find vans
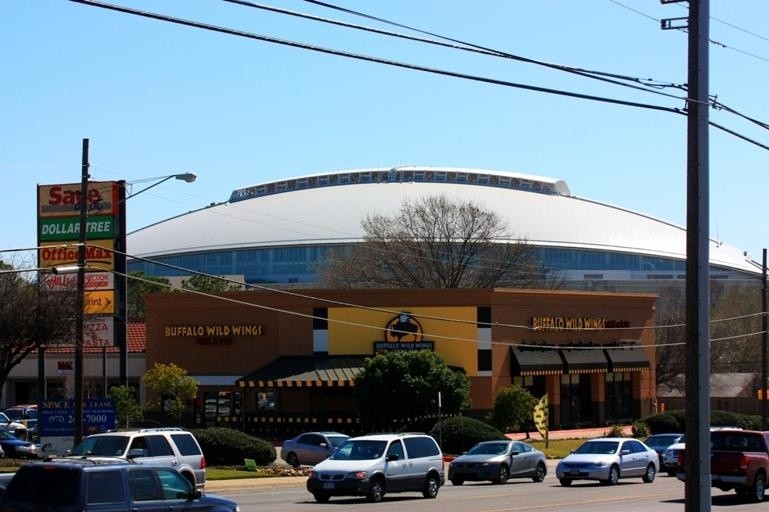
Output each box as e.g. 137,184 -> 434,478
49,426 -> 206,498
662,426 -> 744,477
0,462 -> 240,512
305,432 -> 445,503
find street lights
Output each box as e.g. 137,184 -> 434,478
73,137 -> 197,451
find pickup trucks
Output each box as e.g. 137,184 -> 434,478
675,428 -> 769,502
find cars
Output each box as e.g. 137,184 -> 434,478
0,405 -> 38,459
280,431 -> 352,467
556,437 -> 659,487
643,433 -> 684,467
448,440 -> 549,485
